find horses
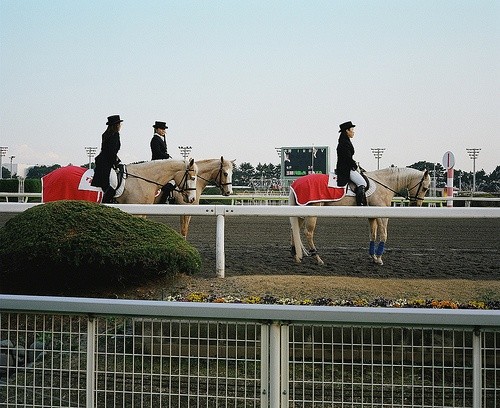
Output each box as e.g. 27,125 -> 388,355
288,167 -> 431,265
41,158 -> 198,218
152,156 -> 236,241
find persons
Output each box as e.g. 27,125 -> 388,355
91,115 -> 123,204
150,121 -> 173,204
336,121 -> 368,206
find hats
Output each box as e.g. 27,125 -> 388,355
338,121 -> 355,133
153,121 -> 168,129
106,115 -> 123,125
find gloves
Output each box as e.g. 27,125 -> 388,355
356,166 -> 360,173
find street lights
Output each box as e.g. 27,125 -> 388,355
275,147 -> 289,179
83,146 -> 98,168
178,146 -> 193,159
371,147 -> 386,170
466,147 -> 482,191
0,146 -> 8,178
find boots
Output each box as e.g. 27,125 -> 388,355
356,185 -> 365,206
159,183 -> 174,204
102,186 -> 116,204
